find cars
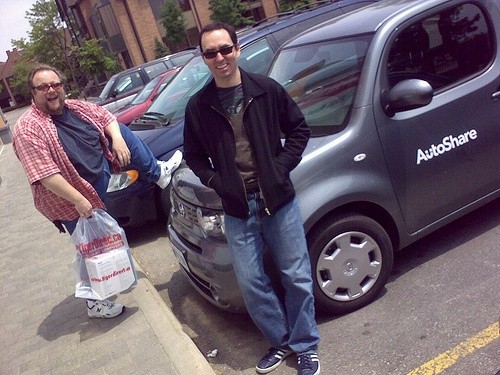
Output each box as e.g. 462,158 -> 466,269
168,0 -> 500,315
104,0 -> 376,230
93,47 -> 208,128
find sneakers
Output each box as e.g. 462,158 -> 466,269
87,300 -> 126,318
297,352 -> 320,375
256,346 -> 295,373
155,150 -> 183,190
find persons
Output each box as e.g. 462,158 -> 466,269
183,22 -> 320,375
12,66 -> 183,320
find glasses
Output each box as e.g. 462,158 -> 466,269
32,81 -> 64,90
203,44 -> 235,59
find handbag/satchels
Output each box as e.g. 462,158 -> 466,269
68,209 -> 137,300
